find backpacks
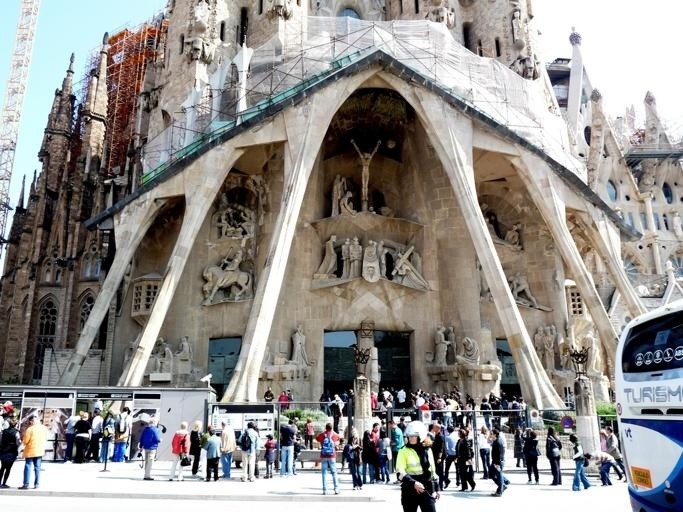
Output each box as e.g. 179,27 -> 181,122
117,413 -> 128,433
321,432 -> 334,456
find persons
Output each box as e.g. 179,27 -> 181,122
170,386 -> 628,498
0,405 -> 163,489
316,236 -> 430,288
434,325 -> 480,364
211,190 -> 258,274
291,325 -> 308,369
506,226 -> 519,244
153,338 -> 168,371
507,274 -> 539,309
173,338 -> 193,360
535,325 -> 565,373
395,420 -> 439,512
330,138 -> 381,220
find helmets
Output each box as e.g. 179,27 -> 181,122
404,420 -> 429,444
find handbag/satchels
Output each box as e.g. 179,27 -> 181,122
583,458 -> 589,467
104,424 -> 114,439
181,457 -> 192,466
241,431 -> 252,451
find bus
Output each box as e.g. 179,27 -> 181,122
614,298 -> 682,512
